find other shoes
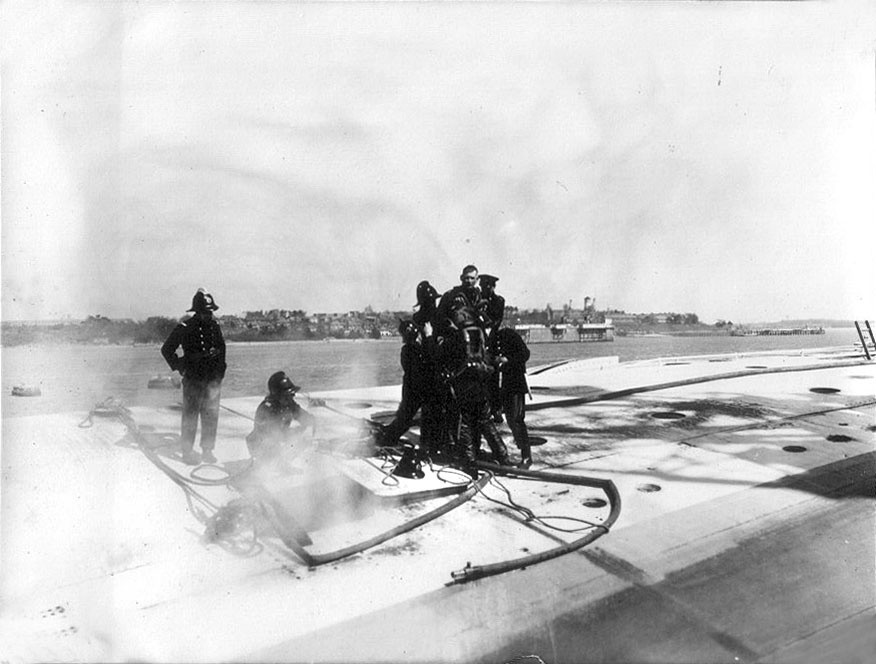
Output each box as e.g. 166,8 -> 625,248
202,448 -> 218,464
367,427 -> 532,480
182,449 -> 202,465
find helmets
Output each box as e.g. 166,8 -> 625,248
186,291 -> 219,312
265,371 -> 301,400
399,281 -> 492,342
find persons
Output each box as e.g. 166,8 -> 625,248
382,265 -> 533,478
245,370 -> 317,474
161,287 -> 227,465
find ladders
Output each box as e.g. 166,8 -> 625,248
855,320 -> 876,360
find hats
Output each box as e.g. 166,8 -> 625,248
478,275 -> 499,288
